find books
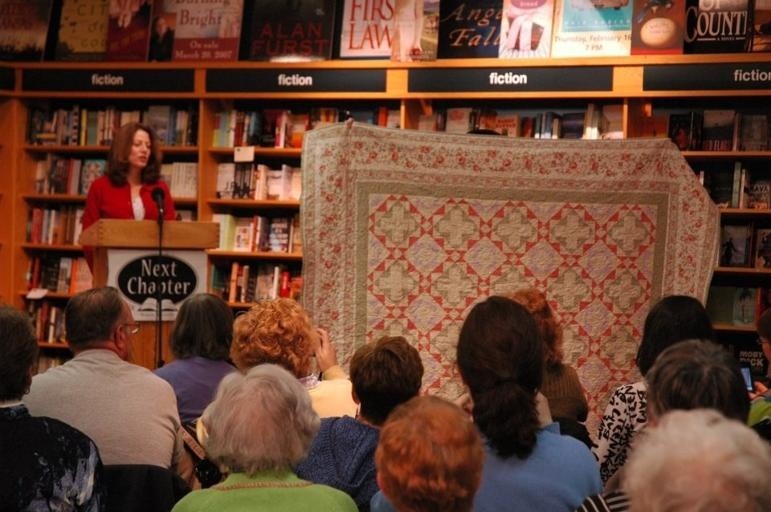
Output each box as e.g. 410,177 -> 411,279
25,102 -> 199,371
663,108 -> 771,389
209,106 -> 401,301
415,105 -> 611,140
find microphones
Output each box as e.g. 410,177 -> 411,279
150,186 -> 167,214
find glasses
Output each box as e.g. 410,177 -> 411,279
119,319 -> 140,334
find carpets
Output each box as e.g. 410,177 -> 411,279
300,123 -> 722,440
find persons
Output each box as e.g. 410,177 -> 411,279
504,288 -> 593,453
146,15 -> 176,63
593,294 -> 716,483
747,318 -> 771,439
375,393 -> 484,510
148,292 -> 239,425
395,0 -> 426,62
575,340 -> 751,510
449,295 -> 603,511
193,299 -> 361,471
28,288 -> 201,486
170,363 -> 357,511
621,407 -> 770,510
500,0 -> 548,50
0,304 -> 104,510
294,337 -> 425,511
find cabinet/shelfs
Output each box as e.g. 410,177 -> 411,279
12,76 -> 199,380
627,91 -> 771,387
403,100 -> 626,142
199,81 -> 401,320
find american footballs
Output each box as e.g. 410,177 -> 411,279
640,19 -> 679,47
